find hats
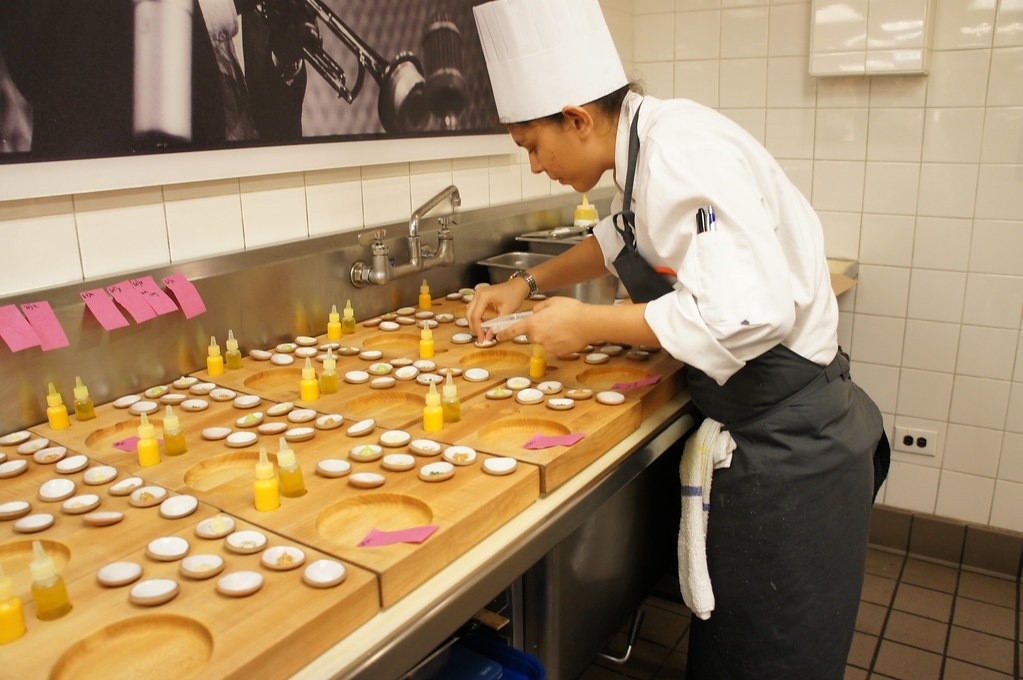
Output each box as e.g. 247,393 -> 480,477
473,0 -> 629,124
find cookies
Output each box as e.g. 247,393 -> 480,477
0,284 -> 648,605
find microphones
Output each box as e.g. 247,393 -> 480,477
419,12 -> 468,118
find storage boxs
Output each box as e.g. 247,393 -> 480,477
432,632 -> 545,680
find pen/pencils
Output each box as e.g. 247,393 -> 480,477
708,204 -> 717,231
696,208 -> 707,234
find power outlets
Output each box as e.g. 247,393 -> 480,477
892,426 -> 938,456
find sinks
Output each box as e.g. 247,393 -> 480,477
476,252 -> 618,305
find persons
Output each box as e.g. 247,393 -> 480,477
465,0 -> 893,680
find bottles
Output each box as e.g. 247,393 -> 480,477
529,344 -> 546,378
342,300 -> 356,334
574,195 -> 599,227
418,279 -> 431,309
46,383 -> 70,430
29,540 -> 71,622
441,371 -> 461,423
206,336 -> 224,376
0,567 -> 27,647
254,447 -> 280,512
419,320 -> 434,360
320,347 -> 340,395
73,375 -> 96,422
422,379 -> 444,433
225,329 -> 242,370
327,304 -> 342,340
136,411 -> 160,467
277,436 -> 306,498
163,404 -> 186,456
300,356 -> 319,402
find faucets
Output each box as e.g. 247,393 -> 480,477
408,186 -> 461,234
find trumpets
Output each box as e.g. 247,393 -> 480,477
246,0 -> 433,135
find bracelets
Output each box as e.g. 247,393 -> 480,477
508,270 -> 538,299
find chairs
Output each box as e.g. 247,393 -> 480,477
594,430 -> 891,666
